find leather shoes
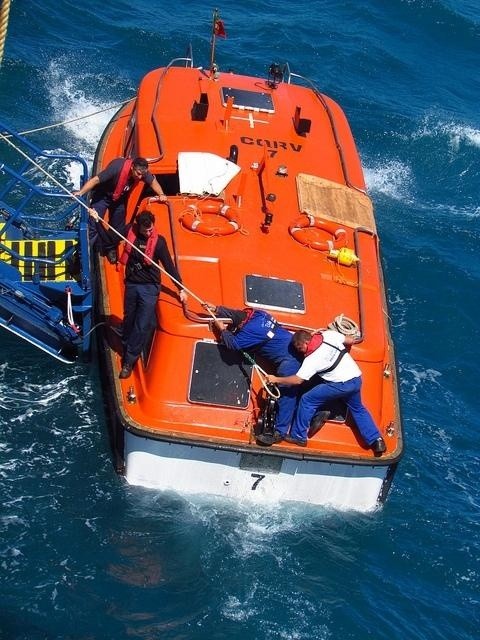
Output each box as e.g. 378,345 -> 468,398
285,434 -> 307,447
373,437 -> 387,454
307,410 -> 331,439
106,248 -> 117,264
119,362 -> 132,379
255,429 -> 280,446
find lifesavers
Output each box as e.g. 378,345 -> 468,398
288,215 -> 347,251
181,202 -> 241,235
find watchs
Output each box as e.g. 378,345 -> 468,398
179,289 -> 185,293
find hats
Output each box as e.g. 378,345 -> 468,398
226,310 -> 247,332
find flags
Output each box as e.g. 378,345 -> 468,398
212,18 -> 228,39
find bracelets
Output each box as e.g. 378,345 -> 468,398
159,194 -> 166,196
214,305 -> 219,313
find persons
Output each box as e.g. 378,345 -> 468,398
262,328 -> 388,456
86,206 -> 189,380
70,156 -> 168,265
200,299 -> 334,446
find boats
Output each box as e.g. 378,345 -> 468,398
86,42 -> 406,520
